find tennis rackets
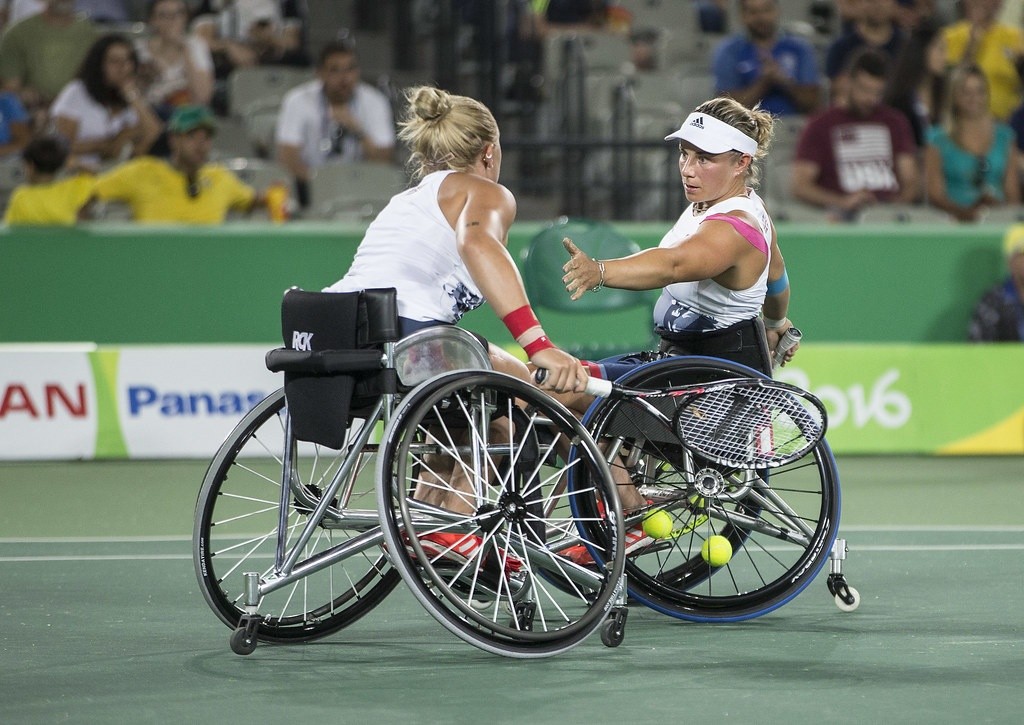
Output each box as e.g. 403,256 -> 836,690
636,325 -> 804,543
533,365 -> 830,471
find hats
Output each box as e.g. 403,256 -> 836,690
166,104 -> 220,135
664,112 -> 758,157
1002,223 -> 1024,258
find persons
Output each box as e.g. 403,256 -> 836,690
319,85 -> 589,577
0,0 -> 1023,346
527,95 -> 798,565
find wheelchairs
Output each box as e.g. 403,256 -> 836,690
544,313 -> 865,620
188,285 -> 635,658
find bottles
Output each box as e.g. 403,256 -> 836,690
266,180 -> 288,224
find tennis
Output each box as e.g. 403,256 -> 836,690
642,507 -> 674,540
700,534 -> 733,568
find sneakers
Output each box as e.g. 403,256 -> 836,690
555,499 -> 656,565
382,519 -> 526,588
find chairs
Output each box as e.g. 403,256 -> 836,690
537,0 -> 1024,226
0,0 -> 408,223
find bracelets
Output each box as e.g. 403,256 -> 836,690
591,257 -> 605,293
761,312 -> 787,328
767,268 -> 790,296
523,335 -> 557,359
501,304 -> 542,342
